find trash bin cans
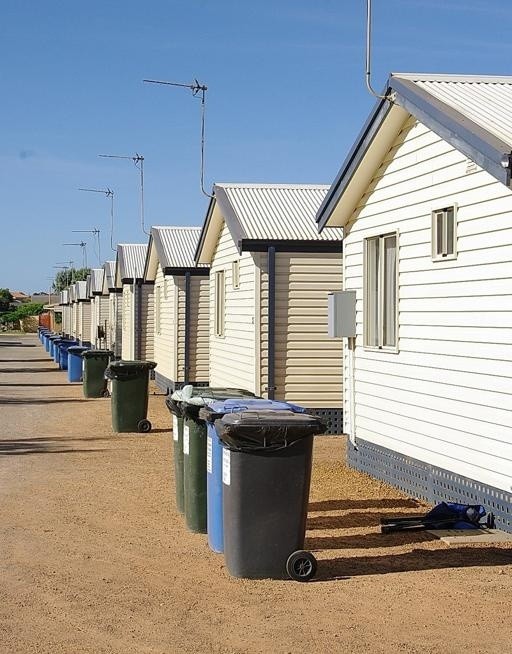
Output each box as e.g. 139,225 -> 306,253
37,325 -> 76,370
68,347 -> 88,382
81,350 -> 113,398
165,387 -> 321,583
108,360 -> 157,433
